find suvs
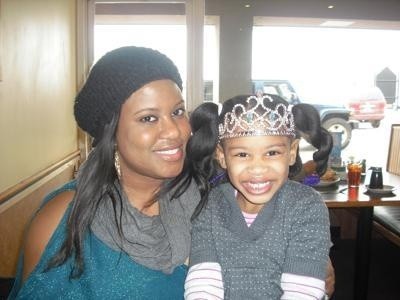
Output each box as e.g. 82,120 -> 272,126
187,79 -> 358,149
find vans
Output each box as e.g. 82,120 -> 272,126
349,84 -> 387,127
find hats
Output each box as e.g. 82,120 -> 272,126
74,46 -> 183,147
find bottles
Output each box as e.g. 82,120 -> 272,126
368,166 -> 383,189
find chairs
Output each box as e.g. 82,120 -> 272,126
365,122 -> 400,252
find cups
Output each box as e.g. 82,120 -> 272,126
346,163 -> 362,188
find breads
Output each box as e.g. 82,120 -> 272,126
304,160 -> 336,181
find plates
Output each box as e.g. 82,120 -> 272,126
313,175 -> 341,188
365,184 -> 397,194
